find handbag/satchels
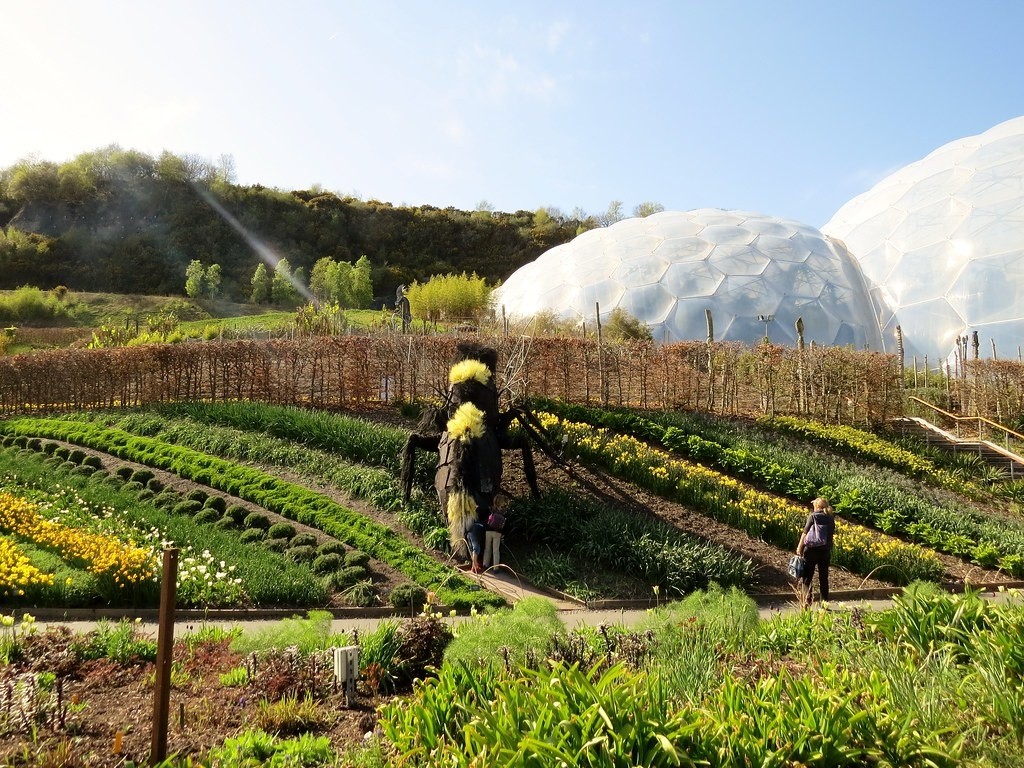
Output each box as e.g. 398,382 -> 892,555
788,555 -> 805,579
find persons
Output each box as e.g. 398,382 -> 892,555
795,497 -> 835,614
465,493 -> 508,574
